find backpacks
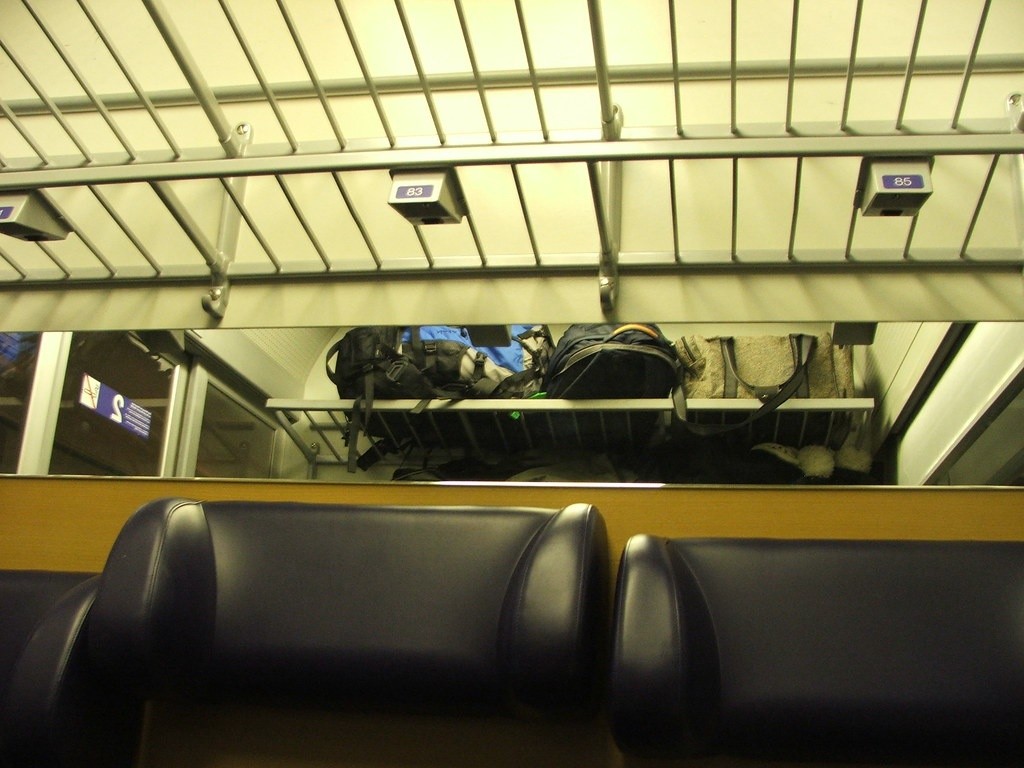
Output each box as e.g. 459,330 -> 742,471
325,324 -> 549,471
540,325 -> 688,461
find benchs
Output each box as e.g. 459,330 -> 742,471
612,528 -> 1024,767
0,570 -> 106,768
71,497 -> 623,766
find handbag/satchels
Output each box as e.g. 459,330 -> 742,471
671,332 -> 855,436
